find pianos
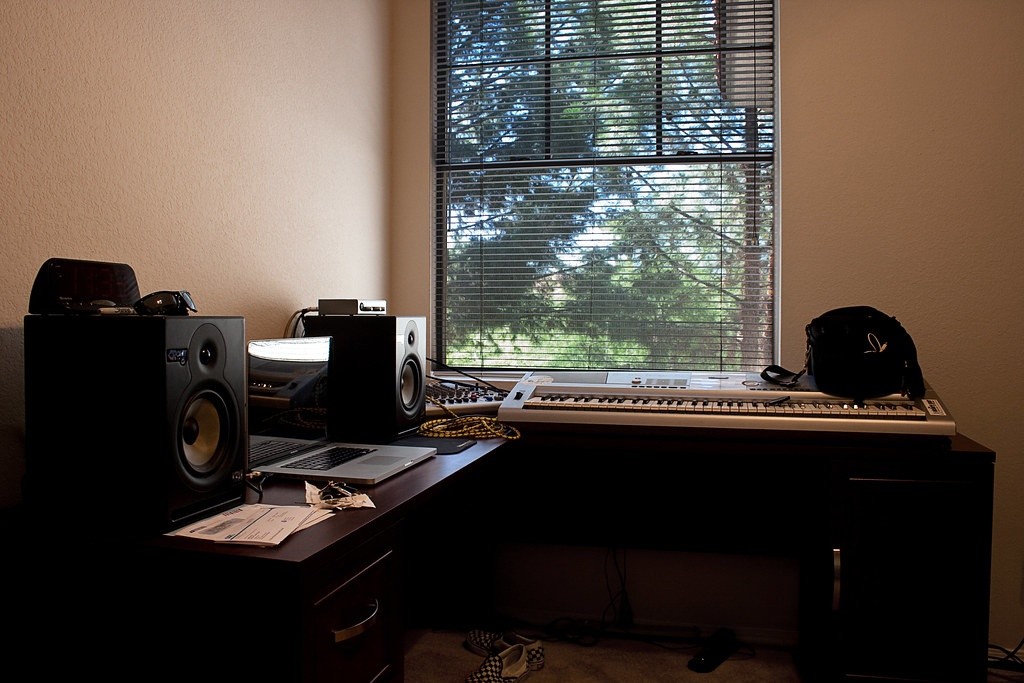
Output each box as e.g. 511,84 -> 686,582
495,366 -> 959,439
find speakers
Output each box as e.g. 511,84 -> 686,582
24,315 -> 248,533
304,315 -> 427,440
25,257 -> 142,314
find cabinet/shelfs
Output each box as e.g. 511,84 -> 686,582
401,434 -> 995,682
0,425 -> 519,683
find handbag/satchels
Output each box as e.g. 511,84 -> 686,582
804,306 -> 925,401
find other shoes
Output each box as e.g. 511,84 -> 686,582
465,644 -> 531,683
466,628 -> 545,671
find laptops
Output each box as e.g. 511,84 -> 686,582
247,336 -> 436,485
